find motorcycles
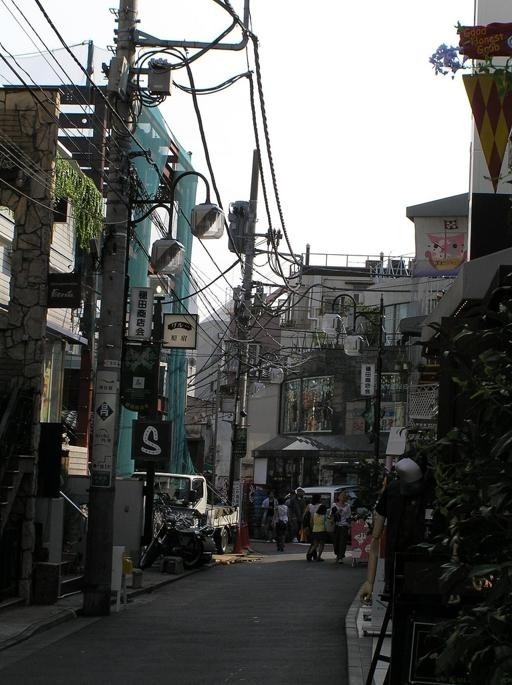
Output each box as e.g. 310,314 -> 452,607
138,496 -> 206,568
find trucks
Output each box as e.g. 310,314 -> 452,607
126,471 -> 240,554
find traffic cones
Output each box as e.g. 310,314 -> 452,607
230,520 -> 252,555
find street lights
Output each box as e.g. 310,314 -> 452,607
320,287 -> 387,538
103,153 -> 227,614
220,347 -> 288,549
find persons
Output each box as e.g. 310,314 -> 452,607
261,491 -> 279,539
248,484 -> 267,539
287,487 -> 354,565
272,496 -> 289,552
359,451 -> 427,626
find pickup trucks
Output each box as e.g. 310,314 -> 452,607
278,483 -> 387,545
